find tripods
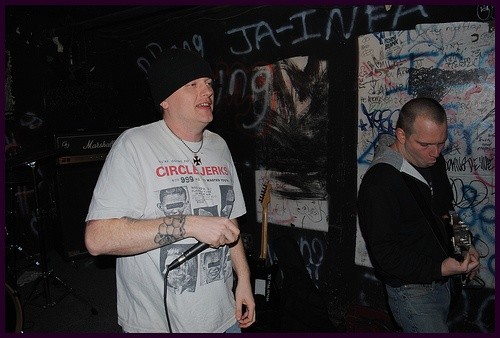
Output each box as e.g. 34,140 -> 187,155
5,149 -> 99,315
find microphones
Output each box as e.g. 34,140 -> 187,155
165,242 -> 210,269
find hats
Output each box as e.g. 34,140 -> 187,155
148,48 -> 214,105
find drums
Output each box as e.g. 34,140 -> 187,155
5,282 -> 24,334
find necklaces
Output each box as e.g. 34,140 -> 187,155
164,118 -> 203,165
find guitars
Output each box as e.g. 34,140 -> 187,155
446,208 -> 472,288
246,180 -> 283,328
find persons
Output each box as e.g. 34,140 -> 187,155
84,48 -> 256,333
358,97 -> 480,333
